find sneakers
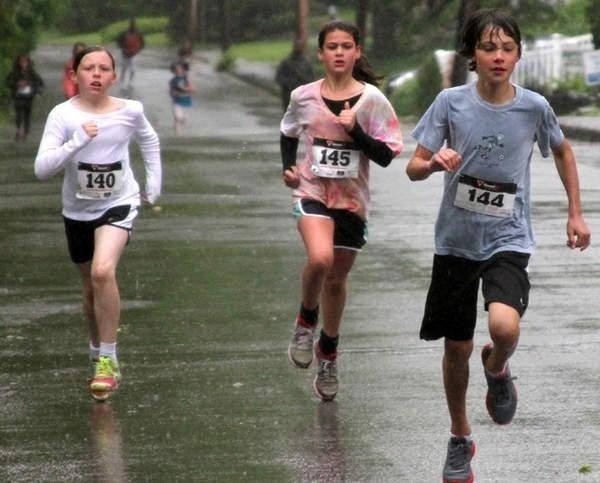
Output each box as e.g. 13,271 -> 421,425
480,344 -> 519,426
313,339 -> 339,403
442,433 -> 477,483
87,355 -> 124,403
287,312 -> 319,369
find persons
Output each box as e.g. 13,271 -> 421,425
276,36 -> 320,135
34,46 -> 163,403
169,63 -> 195,138
280,20 -> 403,403
405,8 -> 591,483
115,15 -> 146,89
64,41 -> 87,98
6,52 -> 45,144
175,36 -> 194,78
